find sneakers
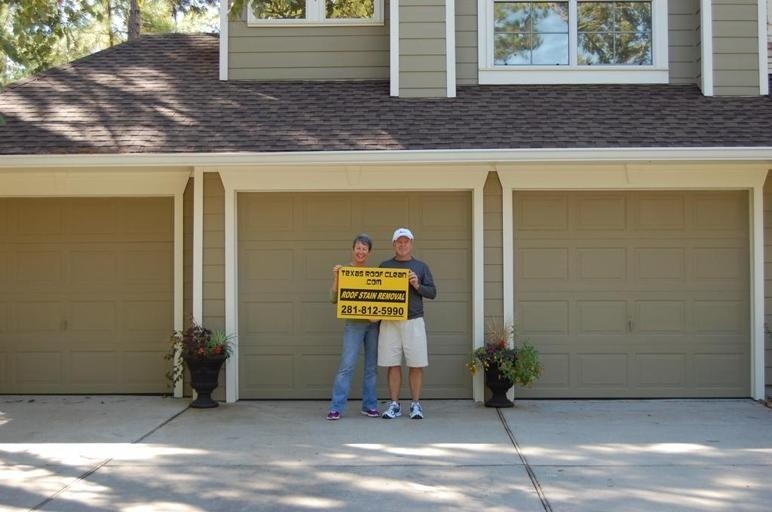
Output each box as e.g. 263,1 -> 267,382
326,411 -> 340,420
382,401 -> 402,419
409,401 -> 423,419
360,408 -> 379,417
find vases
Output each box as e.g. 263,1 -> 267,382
484,362 -> 515,408
184,353 -> 226,408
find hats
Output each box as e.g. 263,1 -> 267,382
392,228 -> 414,244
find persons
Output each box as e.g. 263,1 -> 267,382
326,236 -> 382,419
377,227 -> 436,418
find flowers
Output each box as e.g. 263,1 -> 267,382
161,312 -> 238,399
464,313 -> 545,392
